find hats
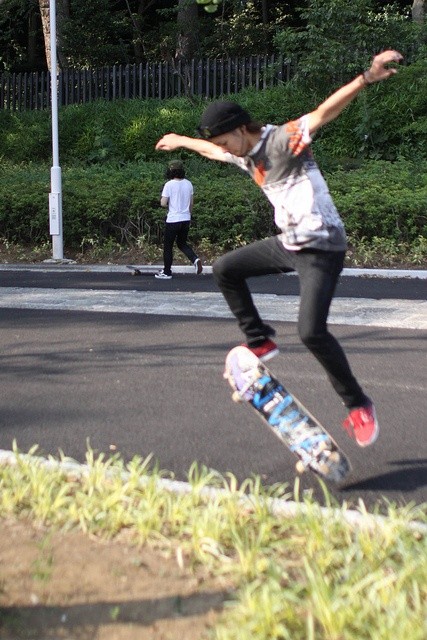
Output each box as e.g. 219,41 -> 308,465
168,160 -> 184,169
197,101 -> 251,139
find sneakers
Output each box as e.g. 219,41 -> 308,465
240,339 -> 281,363
155,269 -> 172,279
194,258 -> 203,275
343,402 -> 380,448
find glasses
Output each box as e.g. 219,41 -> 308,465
197,116 -> 232,139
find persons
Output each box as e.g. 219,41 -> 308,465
155,161 -> 203,279
154,50 -> 403,447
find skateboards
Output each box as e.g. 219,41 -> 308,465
126,266 -> 172,276
222,345 -> 353,491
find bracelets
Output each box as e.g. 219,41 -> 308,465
361,72 -> 371,86
